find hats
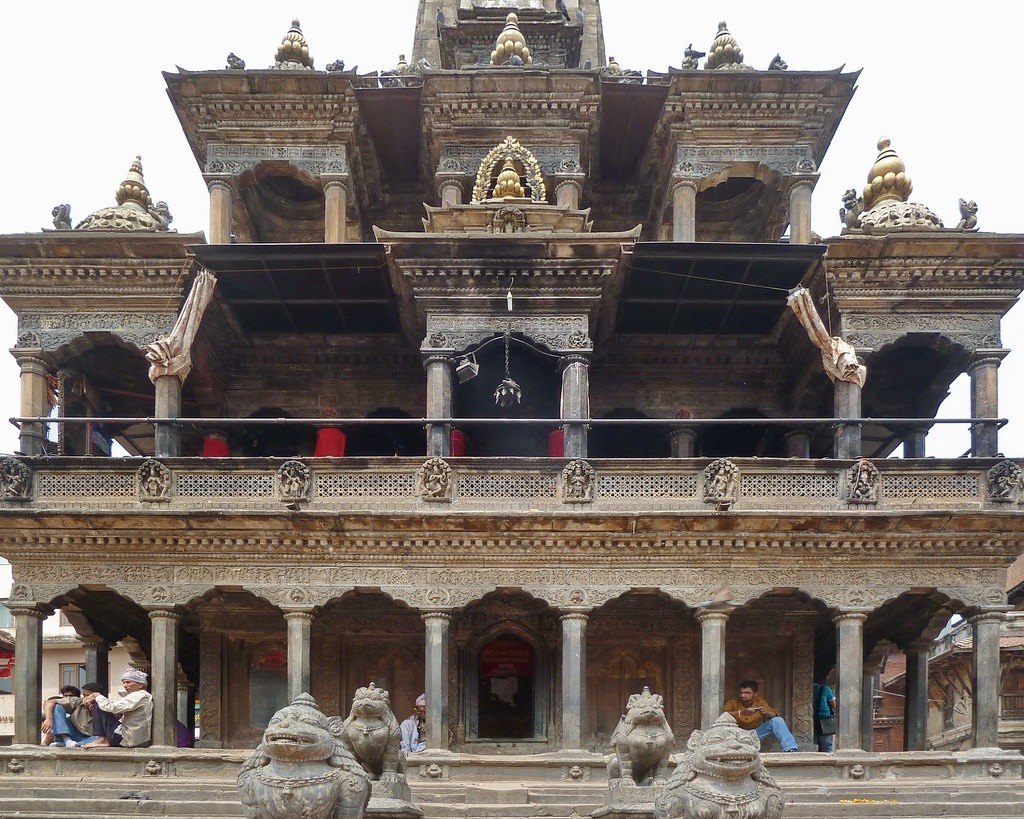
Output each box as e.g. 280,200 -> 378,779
120,669 -> 148,686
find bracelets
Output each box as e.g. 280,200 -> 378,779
738,710 -> 740,716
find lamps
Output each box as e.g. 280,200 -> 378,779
455,353 -> 480,384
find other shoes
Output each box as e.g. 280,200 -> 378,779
787,748 -> 797,752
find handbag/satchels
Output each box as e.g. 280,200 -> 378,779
813,715 -> 839,736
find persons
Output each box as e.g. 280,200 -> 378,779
398,694 -> 452,753
41,669 -> 195,748
813,664 -> 837,752
723,680 -> 798,752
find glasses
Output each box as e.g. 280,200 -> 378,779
739,691 -> 752,694
